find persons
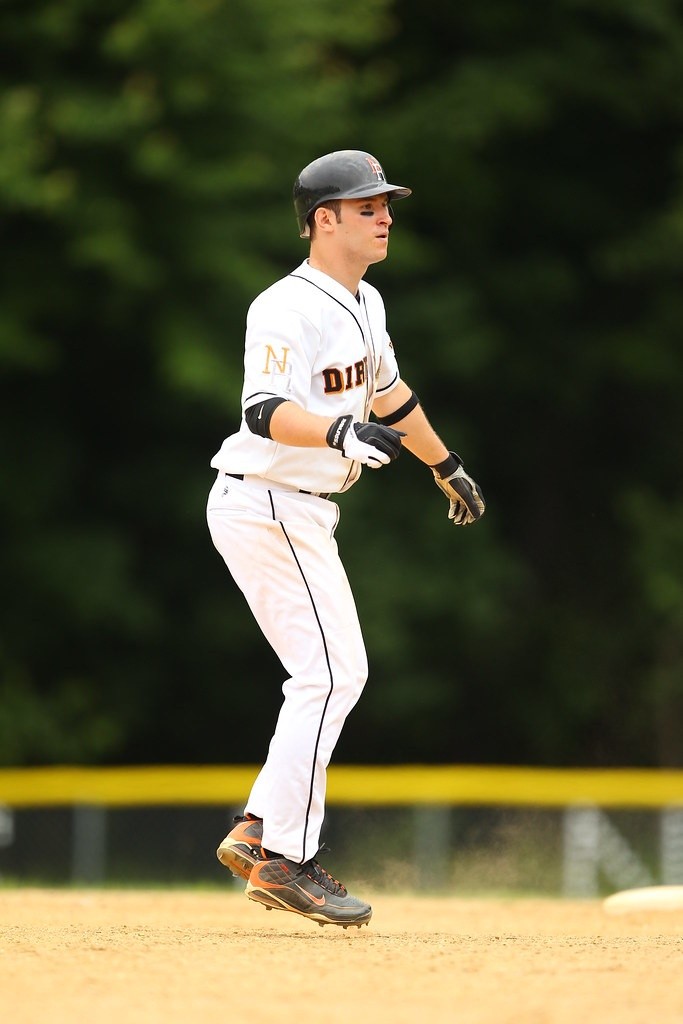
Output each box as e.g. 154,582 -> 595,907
207,148 -> 487,930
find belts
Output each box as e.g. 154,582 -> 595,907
221,471 -> 331,502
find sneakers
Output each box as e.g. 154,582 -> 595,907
217,814 -> 372,930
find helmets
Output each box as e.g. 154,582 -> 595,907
292,150 -> 413,240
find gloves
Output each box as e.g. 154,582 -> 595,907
428,451 -> 486,524
326,415 -> 408,470
